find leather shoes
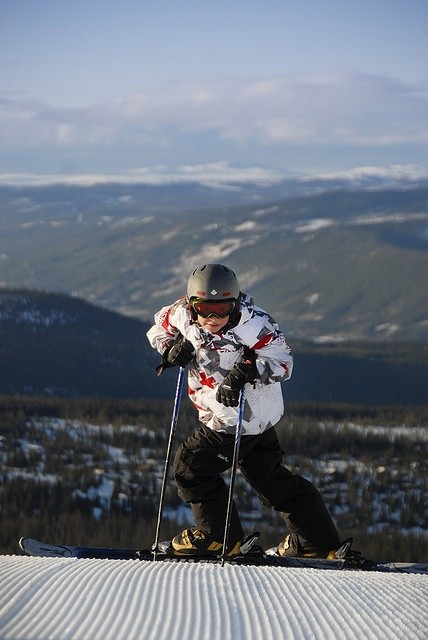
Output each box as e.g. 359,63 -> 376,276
166,341 -> 194,368
216,362 -> 261,408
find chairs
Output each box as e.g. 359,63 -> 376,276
152,528 -> 244,557
265,536 -> 339,560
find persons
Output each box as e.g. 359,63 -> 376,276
146,263 -> 341,559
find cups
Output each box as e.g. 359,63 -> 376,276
189,298 -> 236,319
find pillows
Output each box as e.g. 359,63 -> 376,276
186,263 -> 240,324
